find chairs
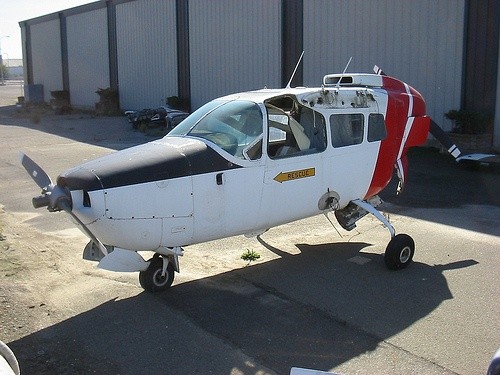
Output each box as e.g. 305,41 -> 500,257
275,146 -> 298,157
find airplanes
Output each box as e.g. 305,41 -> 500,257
19,50 -> 462,295
